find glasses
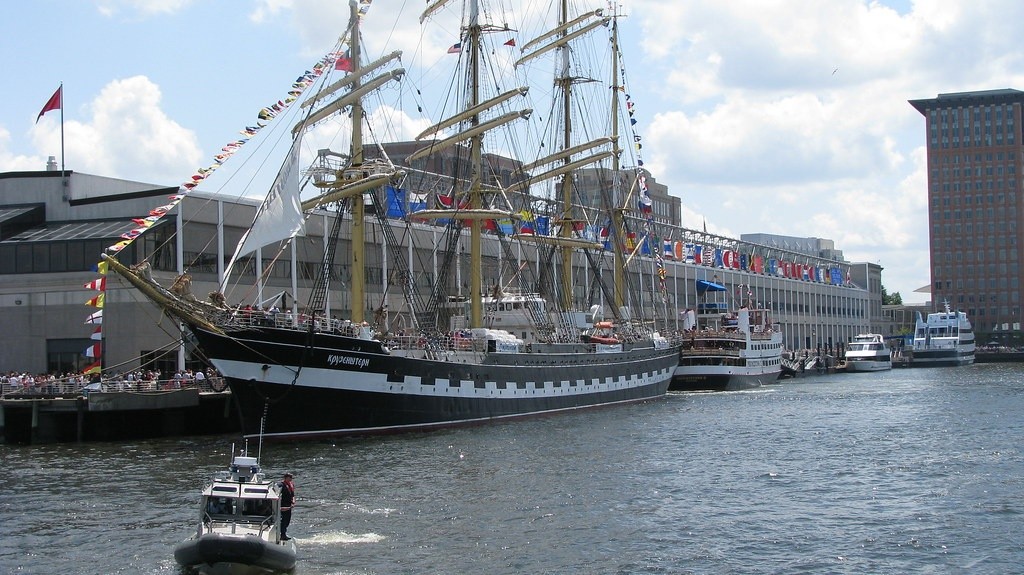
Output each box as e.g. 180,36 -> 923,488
289,476 -> 292,478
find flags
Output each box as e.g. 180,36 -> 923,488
82,292 -> 104,359
503,37 -> 515,46
447,43 -> 461,54
35,85 -> 61,124
82,360 -> 101,375
81,0 -> 851,292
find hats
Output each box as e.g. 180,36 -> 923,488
287,310 -> 291,312
284,473 -> 293,477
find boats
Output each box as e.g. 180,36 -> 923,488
668,268 -> 784,393
844,329 -> 893,373
888,296 -> 976,368
174,417 -> 298,575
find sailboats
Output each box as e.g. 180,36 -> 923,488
100,1 -> 685,448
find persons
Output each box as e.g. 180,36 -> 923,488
280,473 -> 295,541
244,304 -> 468,351
0,367 -> 219,399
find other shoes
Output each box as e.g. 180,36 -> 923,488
280,535 -> 291,540
